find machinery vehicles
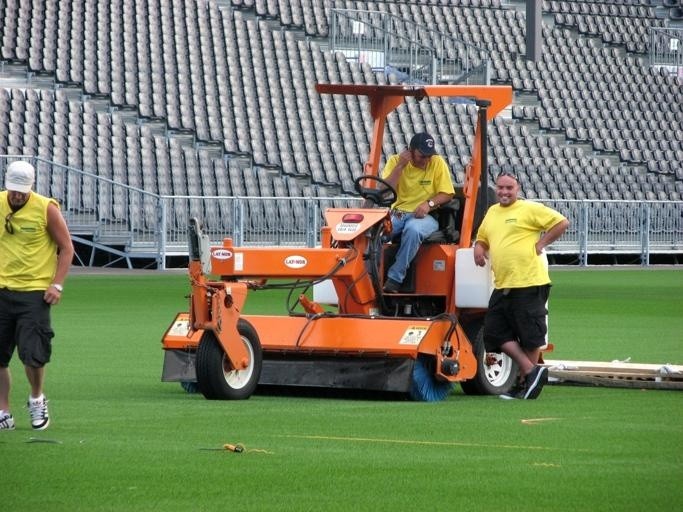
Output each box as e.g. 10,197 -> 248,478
159,83 -> 523,402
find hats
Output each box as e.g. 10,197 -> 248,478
3,160 -> 34,194
408,132 -> 438,158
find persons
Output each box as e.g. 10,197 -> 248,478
473,172 -> 570,401
364,132 -> 457,294
0,160 -> 75,432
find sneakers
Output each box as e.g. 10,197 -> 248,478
499,371 -> 526,401
0,410 -> 15,432
24,394 -> 50,430
522,364 -> 548,400
383,278 -> 402,293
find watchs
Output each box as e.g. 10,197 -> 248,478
427,199 -> 435,209
51,283 -> 63,292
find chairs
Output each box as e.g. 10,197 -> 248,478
0,0 -> 683,244
